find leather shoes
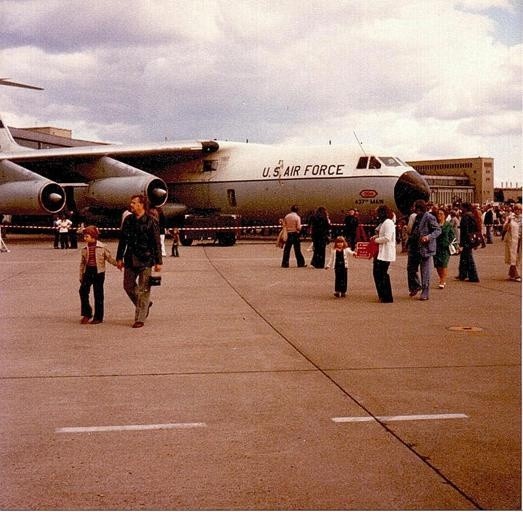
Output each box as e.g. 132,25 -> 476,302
421,292 -> 429,301
145,301 -> 153,318
132,321 -> 144,328
90,319 -> 103,324
79,314 -> 93,324
409,286 -> 422,296
438,284 -> 444,289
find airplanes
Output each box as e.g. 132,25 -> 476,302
0,78 -> 432,247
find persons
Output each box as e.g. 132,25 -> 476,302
276,195 -> 523,302
53,194 -> 182,327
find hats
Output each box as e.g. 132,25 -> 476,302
82,225 -> 101,237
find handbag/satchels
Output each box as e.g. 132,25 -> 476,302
449,237 -> 458,256
148,265 -> 161,285
